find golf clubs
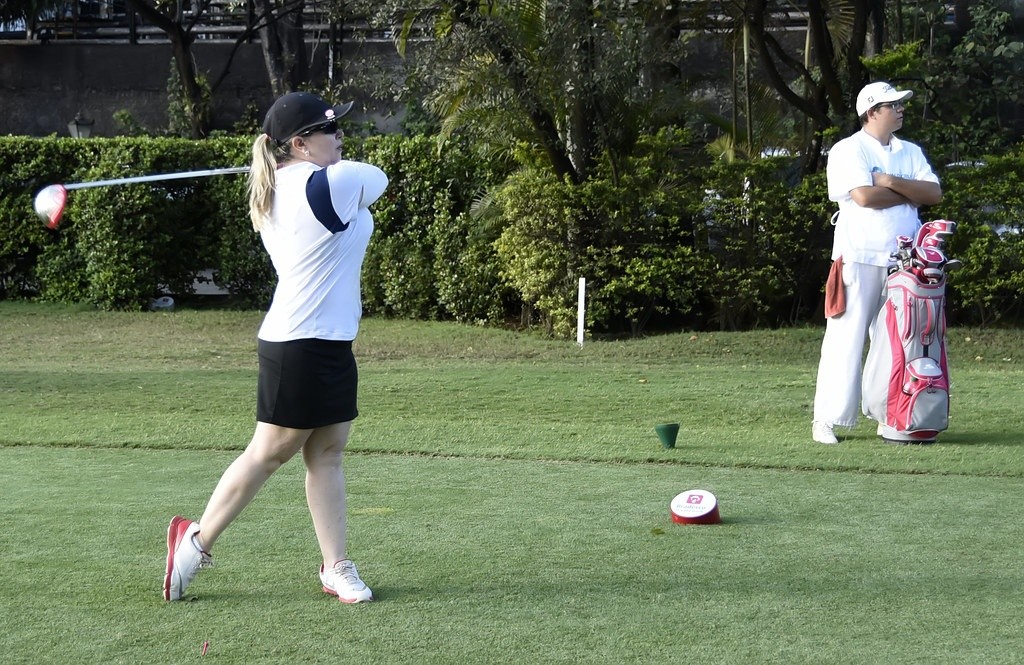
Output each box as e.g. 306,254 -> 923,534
34,165 -> 250,227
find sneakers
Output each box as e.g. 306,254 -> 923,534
877,424 -> 886,436
319,561 -> 373,603
163,516 -> 213,601
812,422 -> 838,445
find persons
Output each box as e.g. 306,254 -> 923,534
162,91 -> 390,604
808,82 -> 942,444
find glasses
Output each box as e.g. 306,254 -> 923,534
873,98 -> 904,110
299,120 -> 338,137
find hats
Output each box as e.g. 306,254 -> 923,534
856,82 -> 914,117
262,92 -> 354,146
